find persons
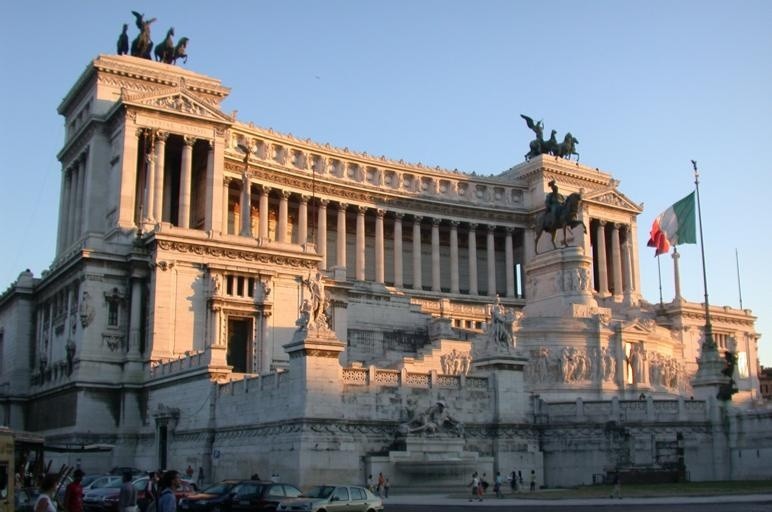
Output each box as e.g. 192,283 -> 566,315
609,469 -> 623,500
522,345 -> 616,385
307,273 -> 325,322
490,295 -> 504,336
439,349 -> 472,376
367,471 -> 390,498
625,344 -> 682,391
251,473 -> 260,492
543,180 -> 565,225
467,467 -> 537,503
396,398 -> 468,437
14,461 -> 205,512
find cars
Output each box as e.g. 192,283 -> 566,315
223,479 -> 303,511
1,466 -> 199,512
275,482 -> 385,511
179,478 -> 244,506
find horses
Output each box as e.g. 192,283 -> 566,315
116,23 -> 129,55
534,192 -> 587,255
174,37 -> 189,66
154,27 -> 175,63
524,129 -> 580,162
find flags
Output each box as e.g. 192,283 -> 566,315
647,191 -> 698,259
